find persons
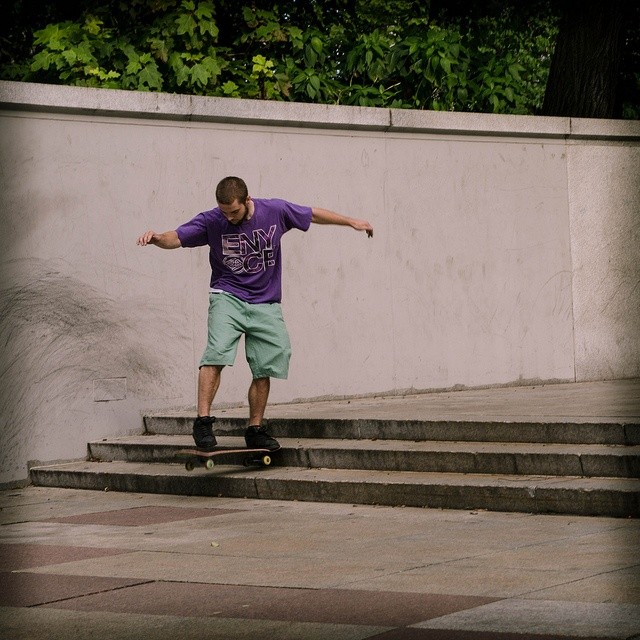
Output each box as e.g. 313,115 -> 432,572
136,177 -> 374,455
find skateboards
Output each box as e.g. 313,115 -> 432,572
175,447 -> 283,470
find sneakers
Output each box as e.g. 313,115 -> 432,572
245,426 -> 279,449
192,415 -> 220,446
